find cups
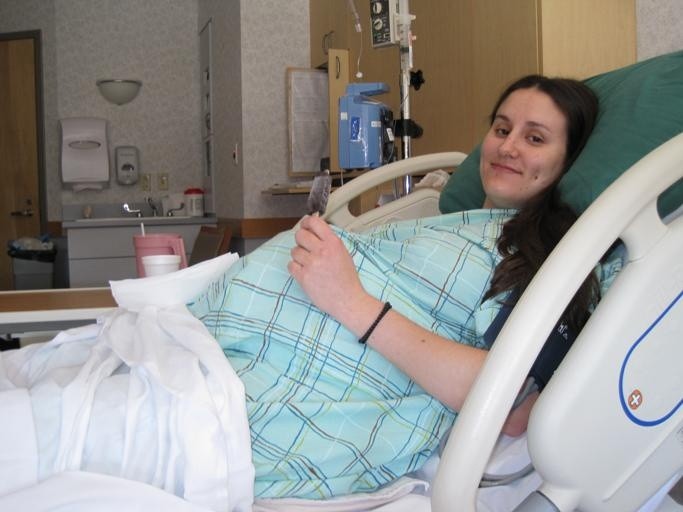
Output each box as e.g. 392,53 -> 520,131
133,233 -> 188,278
142,254 -> 182,277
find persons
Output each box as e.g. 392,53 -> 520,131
2,75 -> 604,502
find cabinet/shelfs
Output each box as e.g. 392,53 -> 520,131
57,225 -> 214,290
262,0 -> 637,219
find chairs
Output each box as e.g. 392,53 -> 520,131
188,224 -> 231,268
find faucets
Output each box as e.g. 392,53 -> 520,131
145,197 -> 157,216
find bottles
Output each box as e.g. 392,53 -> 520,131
184,188 -> 204,217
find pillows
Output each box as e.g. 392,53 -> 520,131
439,45 -> 681,249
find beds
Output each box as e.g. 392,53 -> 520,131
0,135 -> 683,512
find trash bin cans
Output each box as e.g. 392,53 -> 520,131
8,246 -> 58,290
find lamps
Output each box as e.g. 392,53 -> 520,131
97,78 -> 141,106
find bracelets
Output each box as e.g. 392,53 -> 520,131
358,301 -> 391,347
